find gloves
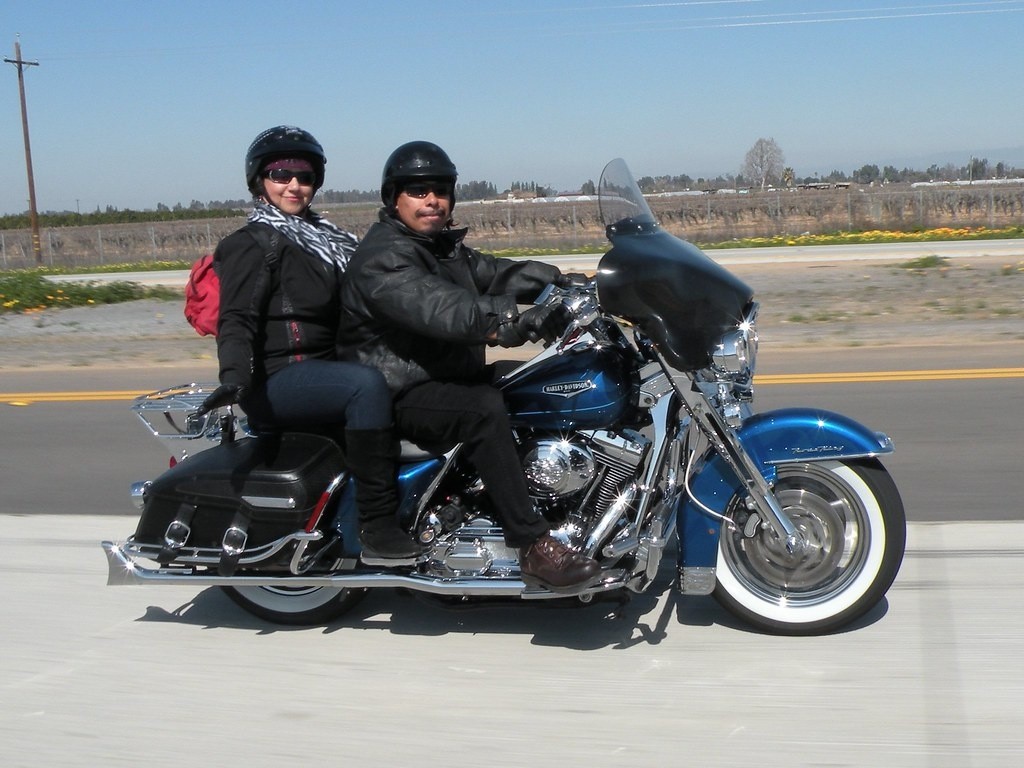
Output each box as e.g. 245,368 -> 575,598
197,385 -> 248,417
496,306 -> 573,349
560,272 -> 587,289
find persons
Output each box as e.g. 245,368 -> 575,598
196,124 -> 420,559
337,140 -> 602,593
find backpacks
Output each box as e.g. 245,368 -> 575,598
183,226 -> 278,336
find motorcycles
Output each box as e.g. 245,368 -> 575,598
102,158 -> 907,639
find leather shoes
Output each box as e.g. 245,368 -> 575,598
519,537 -> 602,592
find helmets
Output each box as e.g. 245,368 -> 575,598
380,141 -> 460,209
245,125 -> 326,198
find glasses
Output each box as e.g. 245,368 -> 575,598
260,167 -> 317,187
398,182 -> 454,199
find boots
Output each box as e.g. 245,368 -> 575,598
345,424 -> 423,559
